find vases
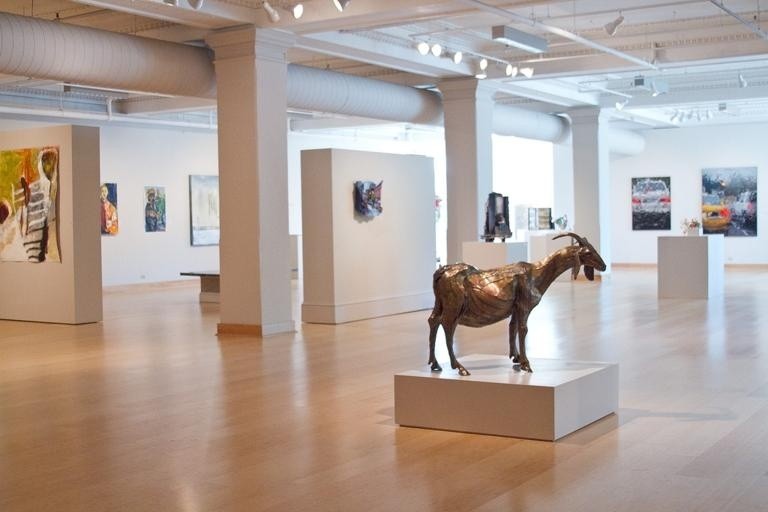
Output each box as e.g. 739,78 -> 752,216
687,227 -> 703,236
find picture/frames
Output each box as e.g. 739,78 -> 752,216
189,173 -> 221,245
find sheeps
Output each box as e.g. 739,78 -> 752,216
428,233 -> 606,376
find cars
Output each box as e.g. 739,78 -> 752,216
702,192 -> 732,235
632,180 -> 671,222
734,189 -> 758,227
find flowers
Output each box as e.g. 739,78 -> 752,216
686,219 -> 701,228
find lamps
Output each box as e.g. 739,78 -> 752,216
616,100 -> 631,112
414,41 -> 534,81
670,109 -> 714,123
604,14 -> 625,38
161,0 -> 204,11
260,0 -> 352,24
738,74 -> 747,88
